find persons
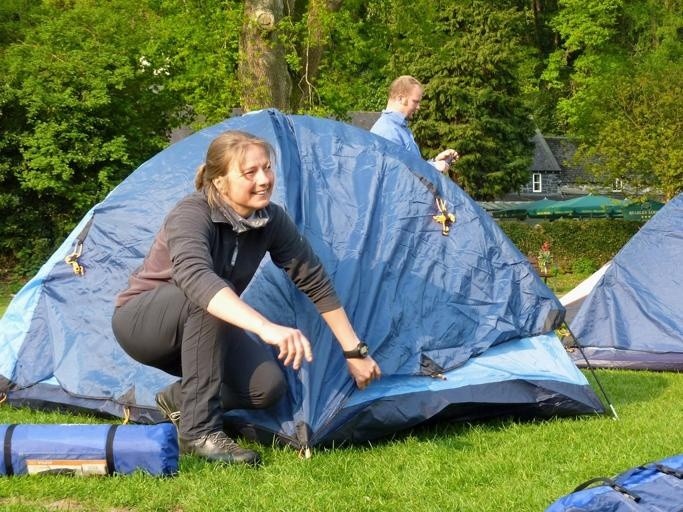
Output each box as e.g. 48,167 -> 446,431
111,128 -> 382,468
368,73 -> 459,176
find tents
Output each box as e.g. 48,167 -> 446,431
0,108 -> 618,459
548,191 -> 682,381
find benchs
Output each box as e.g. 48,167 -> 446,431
528,256 -> 553,284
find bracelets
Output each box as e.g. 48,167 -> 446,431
431,155 -> 437,163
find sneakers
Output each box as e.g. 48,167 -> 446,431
154,391 -> 261,468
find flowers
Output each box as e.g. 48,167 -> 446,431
538,241 -> 552,266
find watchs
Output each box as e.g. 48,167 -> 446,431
340,342 -> 368,362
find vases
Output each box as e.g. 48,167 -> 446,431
538,266 -> 550,272
